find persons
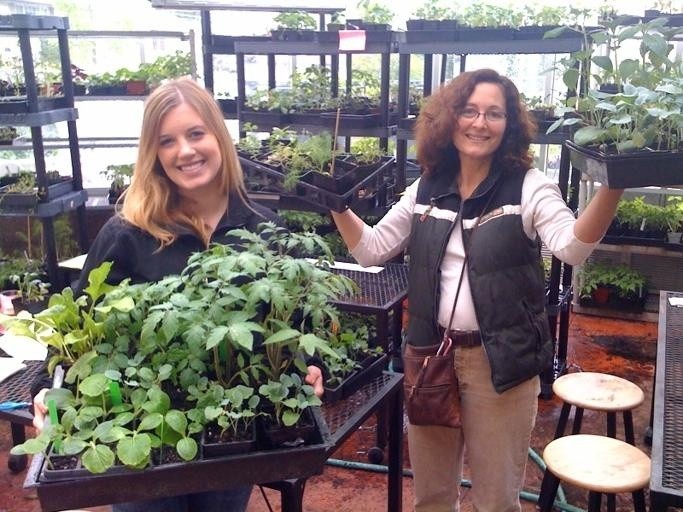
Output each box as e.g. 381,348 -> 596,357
29,81 -> 332,512
330,68 -> 625,512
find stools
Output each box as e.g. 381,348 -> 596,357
536,434 -> 650,512
552,371 -> 645,450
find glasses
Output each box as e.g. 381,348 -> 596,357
461,109 -> 508,121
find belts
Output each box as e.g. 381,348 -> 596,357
438,324 -> 482,348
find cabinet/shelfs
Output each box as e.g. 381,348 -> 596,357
390,38 -> 591,399
234,39 -> 403,266
199,11 -> 343,172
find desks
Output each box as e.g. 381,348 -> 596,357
644,276 -> 682,512
310,262 -> 409,464
0,369 -> 404,512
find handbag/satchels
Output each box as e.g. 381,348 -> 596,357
402,342 -> 463,428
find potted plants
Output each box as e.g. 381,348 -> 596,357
0,170 -> 73,206
575,257 -> 648,309
404,3 -> 599,31
597,0 -> 683,18
97,161 -> 137,204
310,315 -> 383,394
0,79 -> 69,102
269,0 -> 404,40
542,5 -> 683,159
594,195 -> 683,243
0,126 -> 20,145
240,63 -> 400,119
69,50 -> 202,96
402,90 -> 556,123
0,218 -> 81,291
230,121 -> 396,210
